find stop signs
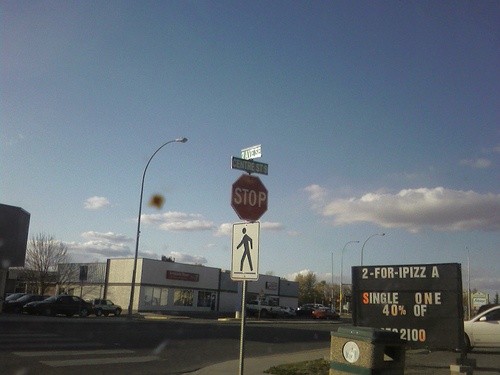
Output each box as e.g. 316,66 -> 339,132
230,173 -> 270,223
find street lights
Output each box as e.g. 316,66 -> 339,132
340,240 -> 360,316
128,136 -> 189,319
361,232 -> 386,266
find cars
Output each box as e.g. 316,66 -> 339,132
248,300 -> 325,317
89,298 -> 123,316
454,305 -> 500,355
5,293 -> 92,317
313,308 -> 340,320
